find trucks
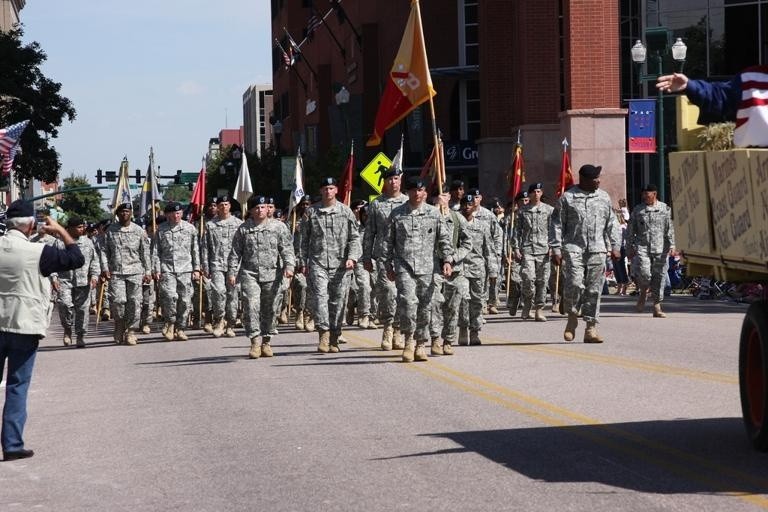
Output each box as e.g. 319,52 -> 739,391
662,145 -> 766,453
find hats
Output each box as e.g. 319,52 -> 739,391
320,177 -> 337,188
527,182 -> 542,193
68,216 -> 85,226
578,164 -> 601,176
265,194 -> 311,218
382,169 -> 449,197
489,191 -> 528,210
163,195 -> 229,212
448,180 -> 480,204
85,219 -> 111,232
115,203 -> 131,214
6,199 -> 33,219
247,195 -> 268,210
641,184 -> 656,192
350,200 -> 364,210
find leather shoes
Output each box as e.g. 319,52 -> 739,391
3,448 -> 33,461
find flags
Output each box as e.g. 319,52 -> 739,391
388,144 -> 405,169
275,0 -> 345,76
507,148 -> 528,197
363,1 -> 440,148
0,120 -> 31,156
419,135 -> 447,187
556,152 -> 575,199
287,153 -> 304,221
233,153 -> 252,213
2,139 -> 21,176
336,157 -> 353,206
623,97 -> 659,154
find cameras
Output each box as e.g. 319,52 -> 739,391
33,218 -> 46,230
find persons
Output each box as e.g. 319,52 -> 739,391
656,66 -> 768,150
29,160 -> 679,366
0,200 -> 85,460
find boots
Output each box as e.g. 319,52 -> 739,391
201,311 -> 242,338
76,335 -> 84,347
136,306 -> 162,334
113,321 -> 126,346
248,336 -> 262,358
317,331 -> 346,353
161,322 -> 188,341
63,328 -> 71,345
563,314 -> 577,341
636,289 -> 646,312
89,305 -> 110,321
652,303 -> 665,318
457,328 -> 468,345
278,306 -> 316,333
479,299 -> 523,315
381,330 -> 453,363
261,337 -> 273,357
522,302 -> 559,322
469,331 -> 480,344
126,329 -> 138,345
583,322 -> 602,343
346,308 -> 379,329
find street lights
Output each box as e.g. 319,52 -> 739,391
630,32 -> 688,296
271,119 -> 283,155
334,86 -> 353,143
232,147 -> 242,177
